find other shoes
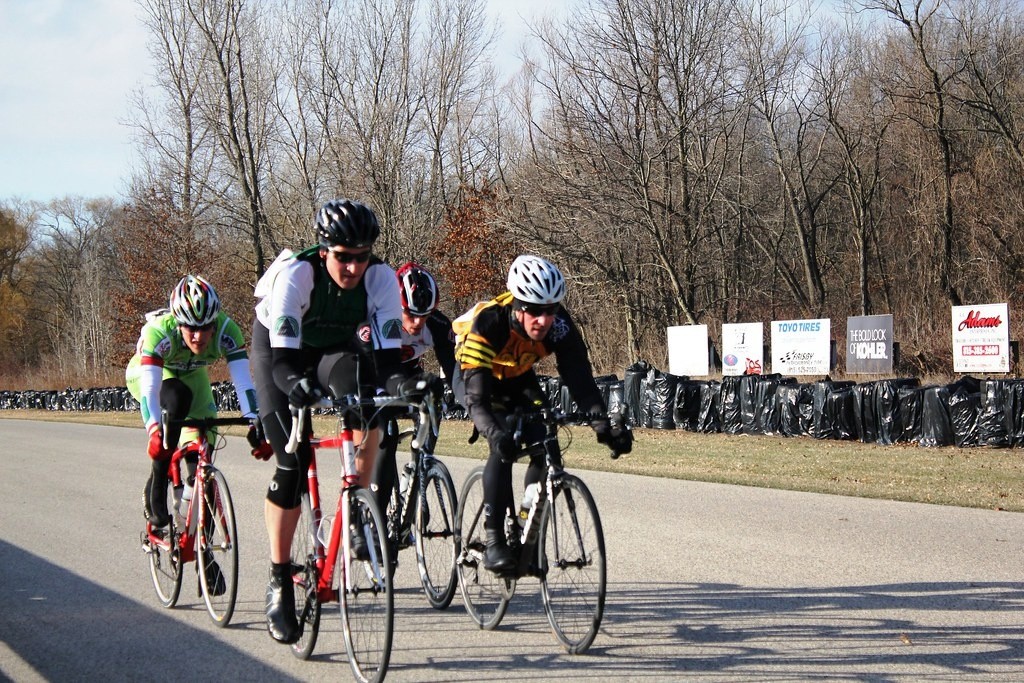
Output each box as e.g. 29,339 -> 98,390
264,562 -> 302,645
522,538 -> 550,577
142,467 -> 171,530
482,525 -> 519,571
343,518 -> 370,561
196,549 -> 226,596
368,524 -> 397,564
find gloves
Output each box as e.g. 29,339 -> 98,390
396,371 -> 445,404
145,427 -> 164,462
286,377 -> 324,409
246,425 -> 277,461
596,422 -> 633,456
488,430 -> 519,465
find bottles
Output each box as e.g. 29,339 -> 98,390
177,473 -> 196,519
517,483 -> 537,528
399,462 -> 412,498
174,480 -> 185,509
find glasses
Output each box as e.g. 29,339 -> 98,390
181,323 -> 214,333
321,241 -> 372,264
523,307 -> 556,317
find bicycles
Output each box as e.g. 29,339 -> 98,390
135,409 -> 261,626
455,401 -> 630,660
342,373 -> 482,610
246,381 -> 445,683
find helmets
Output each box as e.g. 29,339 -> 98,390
316,198 -> 380,248
506,255 -> 566,312
169,275 -> 220,327
395,263 -> 440,319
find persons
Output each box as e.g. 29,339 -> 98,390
451,254 -> 633,572
126,274 -> 274,596
250,198 -> 444,647
337,260 -> 457,557
